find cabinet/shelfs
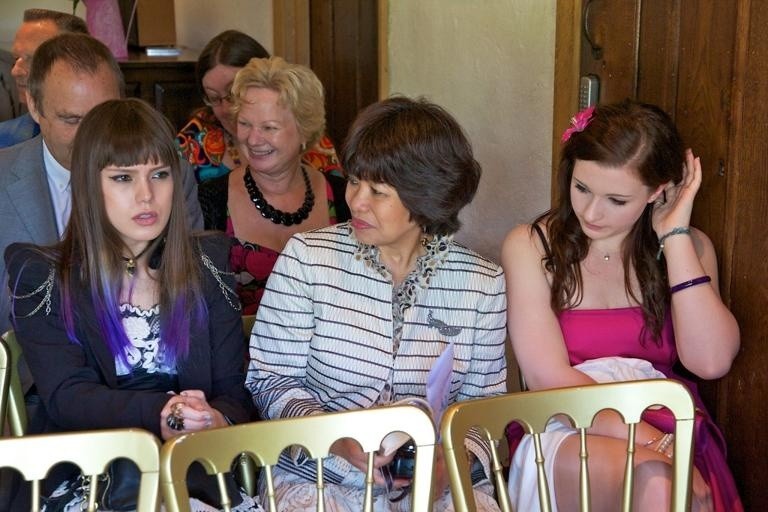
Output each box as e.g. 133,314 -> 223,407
272,1 -> 389,149
551,1 -> 767,511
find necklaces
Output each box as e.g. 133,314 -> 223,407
604,255 -> 609,261
119,238 -> 160,276
501,99 -> 740,511
242,165 -> 316,227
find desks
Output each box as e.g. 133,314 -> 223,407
0,44 -> 201,125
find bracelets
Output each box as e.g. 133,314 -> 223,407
655,228 -> 692,261
643,432 -> 674,460
669,275 -> 711,293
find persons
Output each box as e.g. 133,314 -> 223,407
0,33 -> 124,337
245,97 -> 508,512
197,58 -> 351,255
174,29 -> 344,180
0,8 -> 88,148
7,98 -> 247,512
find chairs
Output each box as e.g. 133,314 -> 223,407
0,329 -> 27,437
160,404 -> 439,512
440,379 -> 697,512
0,428 -> 163,512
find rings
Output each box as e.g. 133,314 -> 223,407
166,414 -> 184,431
170,401 -> 185,417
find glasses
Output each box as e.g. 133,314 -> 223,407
202,92 -> 235,107
